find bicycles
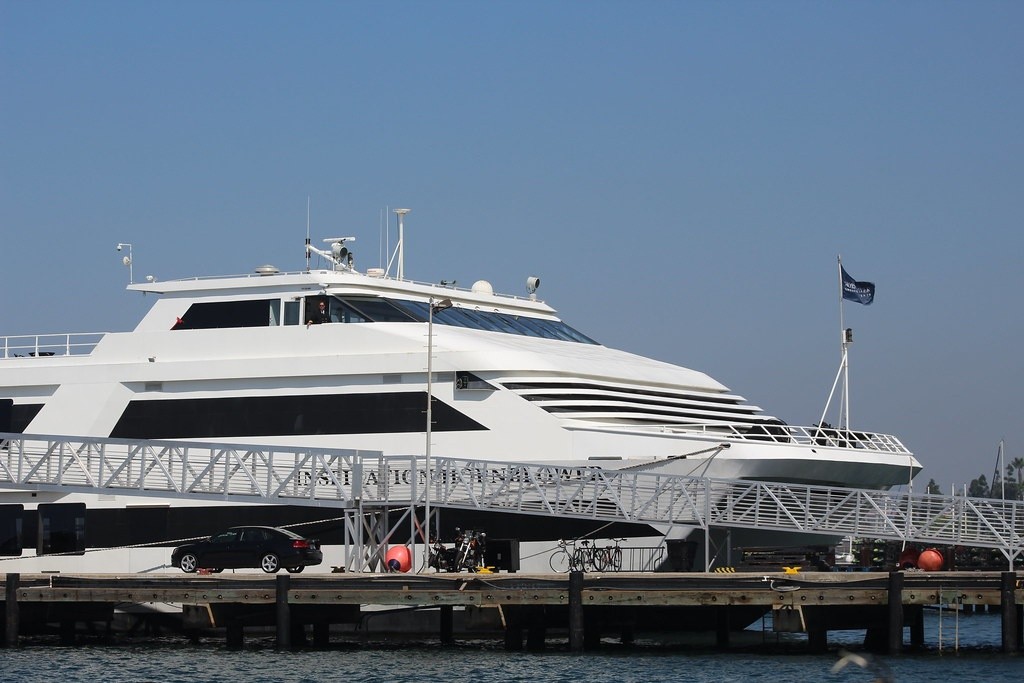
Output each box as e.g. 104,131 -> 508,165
550,534 -> 627,573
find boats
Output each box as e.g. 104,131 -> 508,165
0,195 -> 923,632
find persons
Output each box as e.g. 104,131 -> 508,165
307,302 -> 332,329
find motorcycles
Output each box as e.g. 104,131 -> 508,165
430,528 -> 486,573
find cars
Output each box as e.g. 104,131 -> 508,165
171,526 -> 323,574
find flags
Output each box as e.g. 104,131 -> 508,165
841,262 -> 876,306
